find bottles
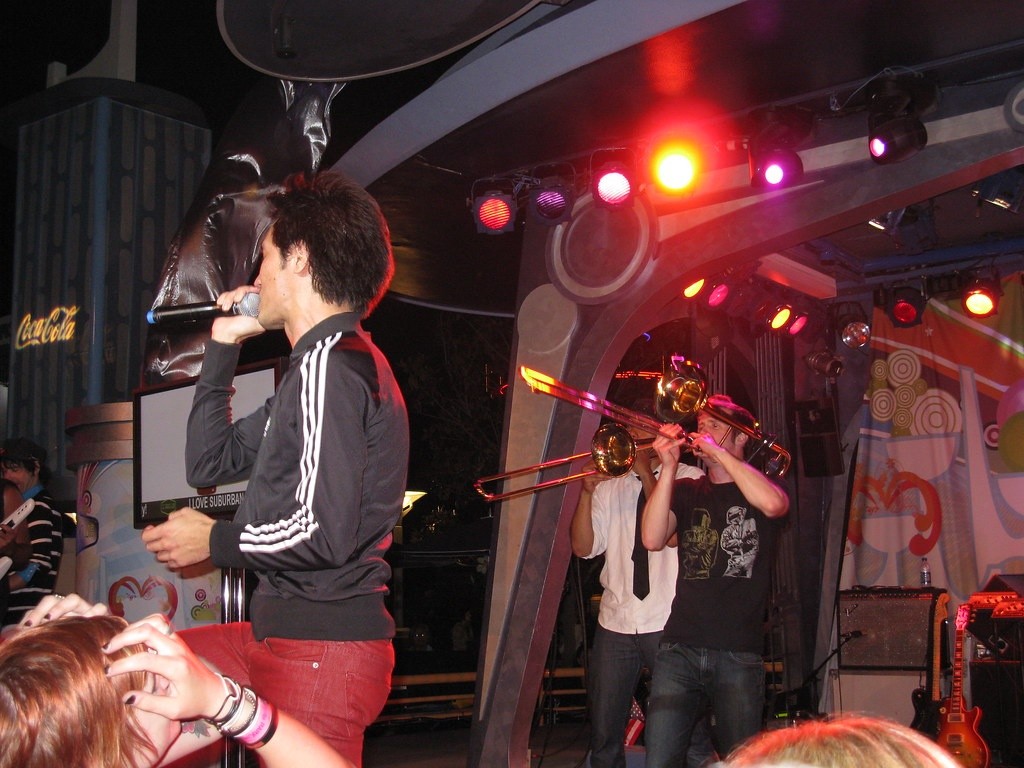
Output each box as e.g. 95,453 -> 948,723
920,557 -> 931,587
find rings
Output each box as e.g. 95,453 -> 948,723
54,594 -> 65,599
165,623 -> 174,636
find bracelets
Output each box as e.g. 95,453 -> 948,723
204,673 -> 278,748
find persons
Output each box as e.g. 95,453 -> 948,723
571,413 -> 715,767
140,166 -> 409,768
0,592 -> 354,768
639,390 -> 790,768
711,710 -> 964,768
0,438 -> 65,641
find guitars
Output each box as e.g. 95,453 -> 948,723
936,603 -> 992,768
906,591 -> 951,743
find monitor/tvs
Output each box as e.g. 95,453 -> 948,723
131,356 -> 290,530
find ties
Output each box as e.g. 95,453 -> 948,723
630,471 -> 658,600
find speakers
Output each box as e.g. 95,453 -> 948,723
793,396 -> 845,478
969,660 -> 1024,768
966,574 -> 1024,660
836,586 -> 949,669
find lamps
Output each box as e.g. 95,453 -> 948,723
589,145 -> 641,212
890,199 -> 940,253
832,241 -> 865,286
834,301 -> 871,349
965,164 -> 1024,215
747,107 -> 816,194
466,174 -> 519,234
680,260 -> 812,337
868,76 -> 935,164
957,265 -> 1006,321
868,212 -> 893,230
805,351 -> 842,378
529,161 -> 575,225
872,276 -> 929,328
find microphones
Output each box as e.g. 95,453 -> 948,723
147,292 -> 260,323
841,630 -> 868,638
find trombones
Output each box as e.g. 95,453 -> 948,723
518,355 -> 792,479
474,422 -> 692,504
